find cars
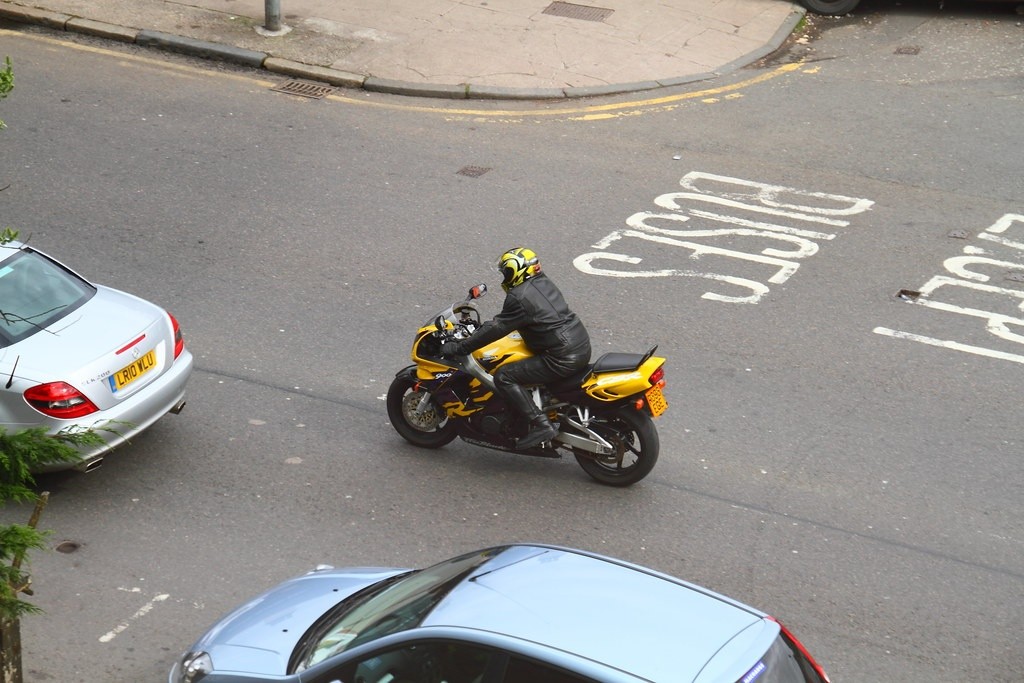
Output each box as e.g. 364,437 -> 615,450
1,236 -> 195,488
171,543 -> 830,683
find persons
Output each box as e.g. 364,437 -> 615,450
443,247 -> 591,450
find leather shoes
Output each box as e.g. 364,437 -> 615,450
515,413 -> 560,451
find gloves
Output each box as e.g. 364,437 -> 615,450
442,342 -> 461,360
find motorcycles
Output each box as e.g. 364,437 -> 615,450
388,282 -> 668,487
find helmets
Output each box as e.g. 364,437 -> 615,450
497,247 -> 542,293
